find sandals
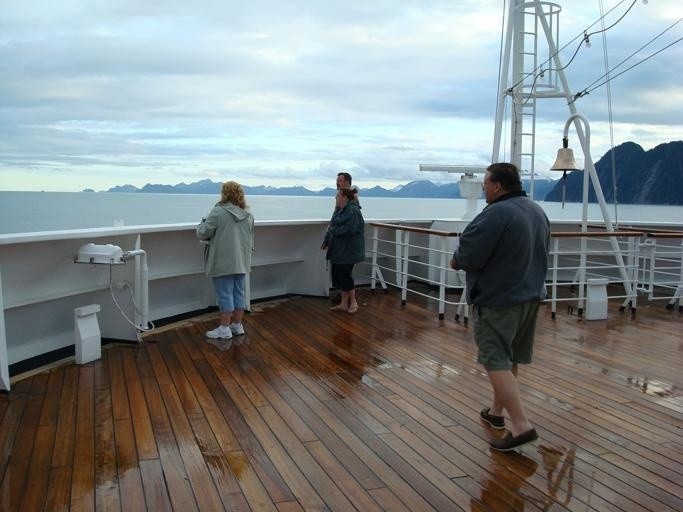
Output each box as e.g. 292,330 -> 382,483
328,292 -> 362,314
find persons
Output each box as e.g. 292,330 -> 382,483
331,172 -> 361,303
450,162 -> 551,452
320,188 -> 366,315
196,181 -> 255,339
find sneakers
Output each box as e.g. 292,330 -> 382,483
205,325 -> 232,340
229,322 -> 244,335
489,428 -> 540,452
479,407 -> 505,430
